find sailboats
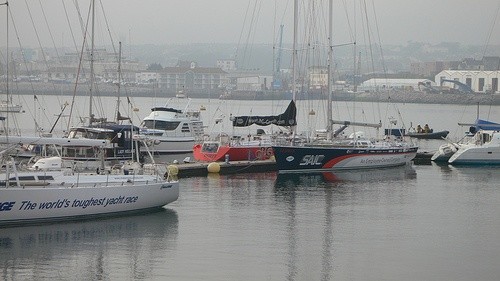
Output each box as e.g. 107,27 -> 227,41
0,0 -> 422,228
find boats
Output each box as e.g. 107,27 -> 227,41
431,102 -> 500,164
406,130 -> 449,141
